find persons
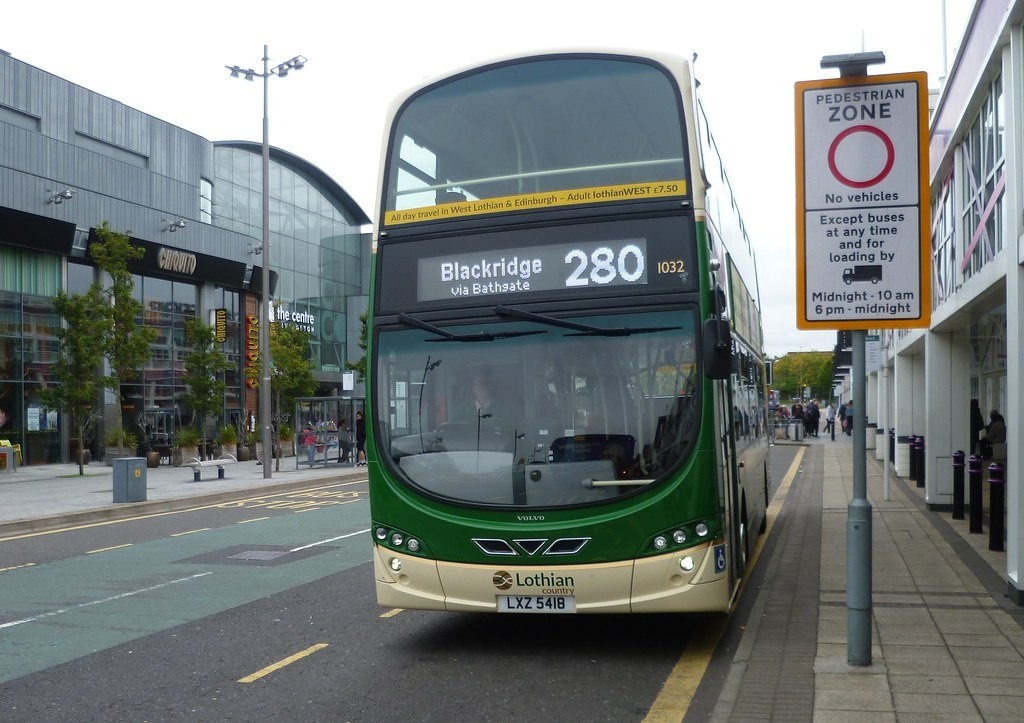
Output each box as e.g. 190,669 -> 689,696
970,399 -> 1006,461
774,397 -> 853,438
734,402 -> 768,441
255,411 -> 366,469
452,372 -> 514,450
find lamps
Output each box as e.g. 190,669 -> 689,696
46,187 -> 77,204
161,218 -> 188,232
249,242 -> 271,256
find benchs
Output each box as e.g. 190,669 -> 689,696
180,454 -> 237,482
315,441 -> 354,453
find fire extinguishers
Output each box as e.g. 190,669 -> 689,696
434,391 -> 450,424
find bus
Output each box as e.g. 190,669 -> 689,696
359,50 -> 773,618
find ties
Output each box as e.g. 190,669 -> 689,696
478,407 -> 484,421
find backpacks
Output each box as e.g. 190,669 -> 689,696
298,429 -> 310,446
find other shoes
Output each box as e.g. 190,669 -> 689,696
256,462 -> 262,465
362,462 -> 367,467
310,465 -> 321,469
357,463 -> 362,467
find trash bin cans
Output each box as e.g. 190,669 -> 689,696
0,440 -> 21,468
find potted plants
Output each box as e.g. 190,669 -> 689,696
273,423 -> 293,458
101,427 -> 139,466
170,427 -> 199,467
230,408 -> 253,461
134,410 -> 164,468
209,424 -> 238,461
70,409 -> 106,465
246,422 -> 263,460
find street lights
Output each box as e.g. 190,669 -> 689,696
225,44 -> 308,479
